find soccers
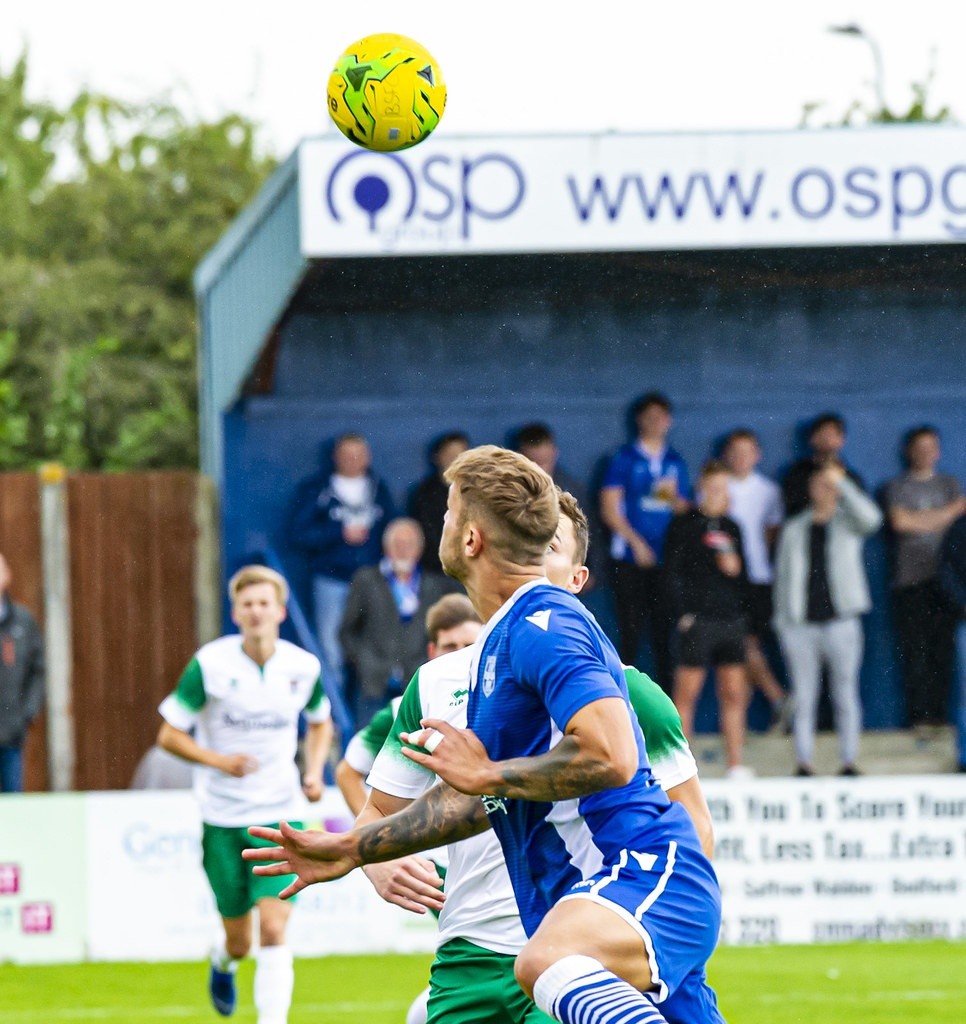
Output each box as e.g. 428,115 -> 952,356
324,29 -> 448,156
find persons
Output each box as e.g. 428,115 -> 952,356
771,461 -> 884,775
599,392 -> 694,669
286,432 -> 396,690
353,486 -> 716,1024
664,470 -> 752,778
408,432 -> 469,575
157,567 -> 334,1024
879,426 -> 966,729
722,426 -> 793,737
782,415 -> 864,515
0,556 -> 45,793
241,445 -> 724,1024
516,418 -> 558,478
337,592 -> 487,917
341,516 -> 438,724
937,512 -> 965,776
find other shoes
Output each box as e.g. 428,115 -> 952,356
726,765 -> 755,782
840,764 -> 858,776
798,765 -> 813,777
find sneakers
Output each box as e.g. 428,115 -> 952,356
210,960 -> 236,1015
